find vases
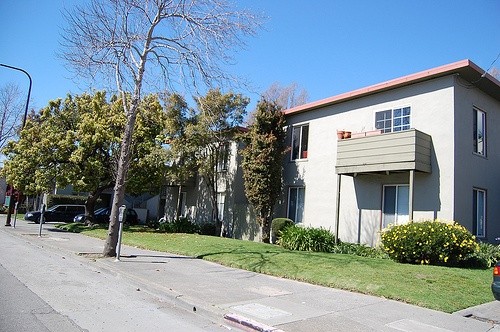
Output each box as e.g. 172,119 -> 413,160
337,131 -> 351,139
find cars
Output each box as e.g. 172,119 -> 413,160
24,205 -> 86,225
73,207 -> 138,226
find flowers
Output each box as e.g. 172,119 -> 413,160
375,218 -> 480,266
